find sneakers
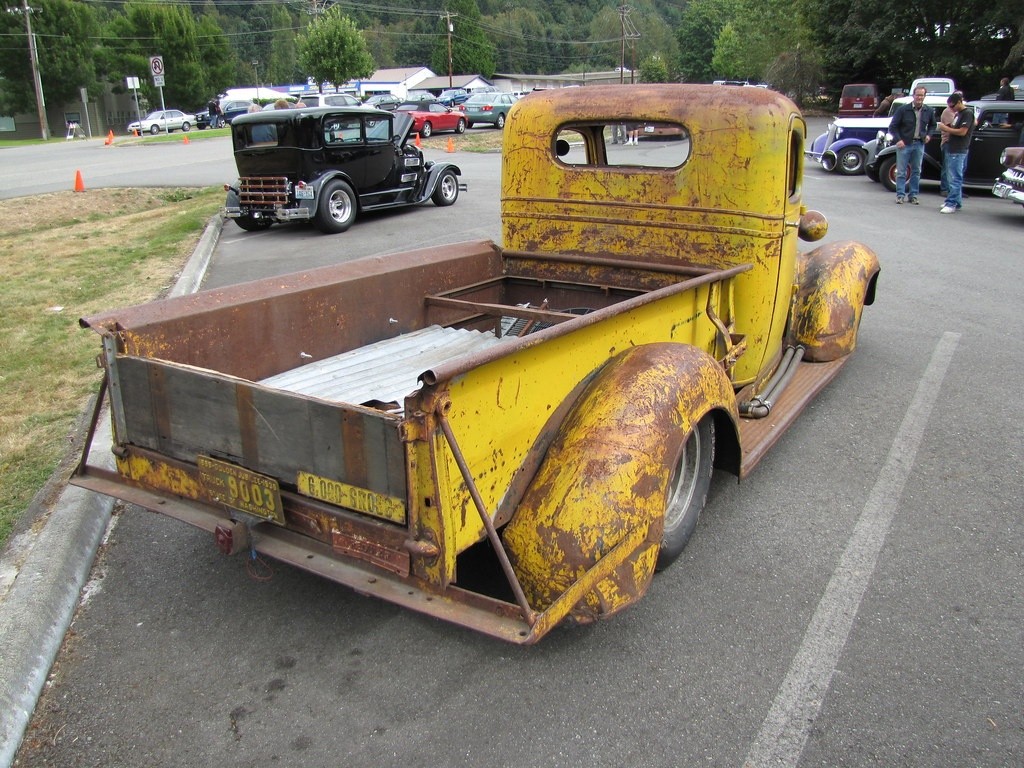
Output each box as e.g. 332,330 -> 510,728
940,203 -> 955,213
896,195 -> 905,204
908,197 -> 919,205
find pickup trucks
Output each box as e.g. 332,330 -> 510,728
902,78 -> 963,97
69,81 -> 882,646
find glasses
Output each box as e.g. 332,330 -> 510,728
949,102 -> 957,108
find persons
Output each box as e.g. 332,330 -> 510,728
999,122 -> 1024,146
888,87 -> 937,204
248,99 -> 306,145
996,78 -> 1015,101
938,90 -> 975,213
610,125 -> 640,145
872,92 -> 906,117
207,97 -> 221,129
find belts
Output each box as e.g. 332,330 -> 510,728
913,139 -> 920,142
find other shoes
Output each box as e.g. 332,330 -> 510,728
210,125 -> 212,128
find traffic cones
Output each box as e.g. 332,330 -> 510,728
415,132 -> 425,150
444,135 -> 456,153
183,136 -> 189,144
72,170 -> 86,191
134,128 -> 138,137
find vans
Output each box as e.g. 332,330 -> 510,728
839,85 -> 881,115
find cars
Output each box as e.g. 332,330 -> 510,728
366,95 -> 402,111
195,100 -> 263,129
127,109 -> 197,136
803,96 -> 966,175
262,102 -> 297,110
391,101 -> 468,138
297,93 -> 374,107
863,100 -> 1024,192
992,147 -> 1024,207
223,104 -> 468,234
404,86 -> 499,108
458,92 -> 520,129
